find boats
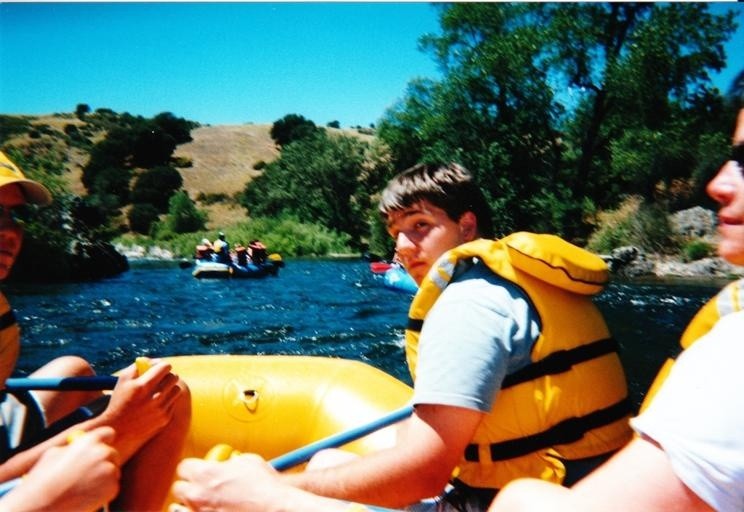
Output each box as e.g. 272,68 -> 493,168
382,264 -> 420,295
99,354 -> 458,512
191,259 -> 280,279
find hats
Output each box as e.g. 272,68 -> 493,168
0,153 -> 56,209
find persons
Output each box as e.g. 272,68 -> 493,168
278,158 -> 635,512
196,231 -> 266,266
0,152 -> 191,512
168,67 -> 744,512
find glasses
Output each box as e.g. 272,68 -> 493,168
1,201 -> 36,230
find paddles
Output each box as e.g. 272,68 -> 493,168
268,251 -> 282,263
371,261 -> 403,274
